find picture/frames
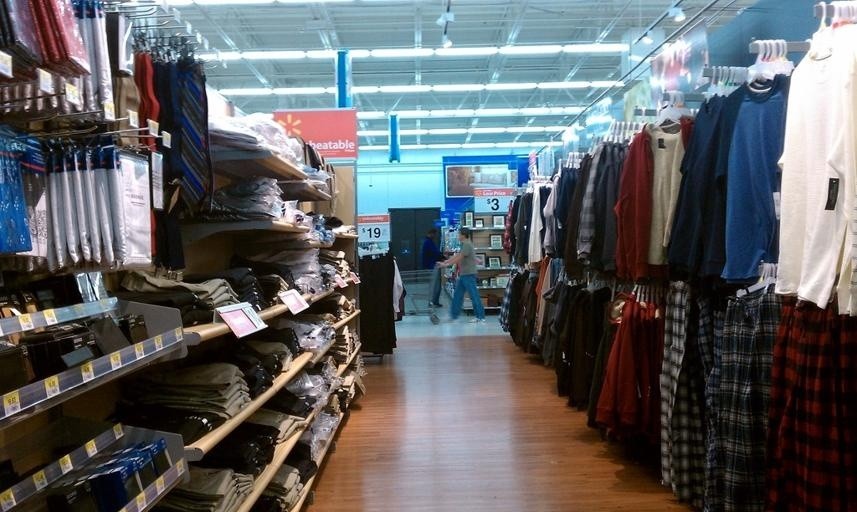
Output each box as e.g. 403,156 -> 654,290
476,255 -> 485,268
497,276 -> 508,286
445,164 -> 508,199
476,219 -> 484,228
492,235 -> 502,250
466,213 -> 473,228
489,258 -> 501,268
494,216 -> 505,230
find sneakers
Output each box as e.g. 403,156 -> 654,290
441,318 -> 460,325
468,317 -> 486,324
428,302 -> 442,308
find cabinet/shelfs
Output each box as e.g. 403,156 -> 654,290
326,163 -> 362,409
310,148 -> 344,465
0,2 -> 185,512
184,78 -> 313,511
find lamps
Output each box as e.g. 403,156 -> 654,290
491,278 -> 497,286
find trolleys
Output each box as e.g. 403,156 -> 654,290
400,262 -> 443,325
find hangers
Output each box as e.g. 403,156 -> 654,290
829,1 -> 857,27
587,269 -> 608,291
641,106 -> 649,136
812,2 -> 827,41
737,263 -> 778,294
654,91 -> 693,130
631,282 -> 666,305
529,173 -> 553,187
558,158 -> 564,178
750,39 -> 787,79
708,62 -> 748,95
567,151 -> 584,169
590,135 -> 601,156
604,119 -> 640,145
361,244 -> 387,259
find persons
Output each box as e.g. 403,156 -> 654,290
436,227 -> 486,324
419,229 -> 447,308
